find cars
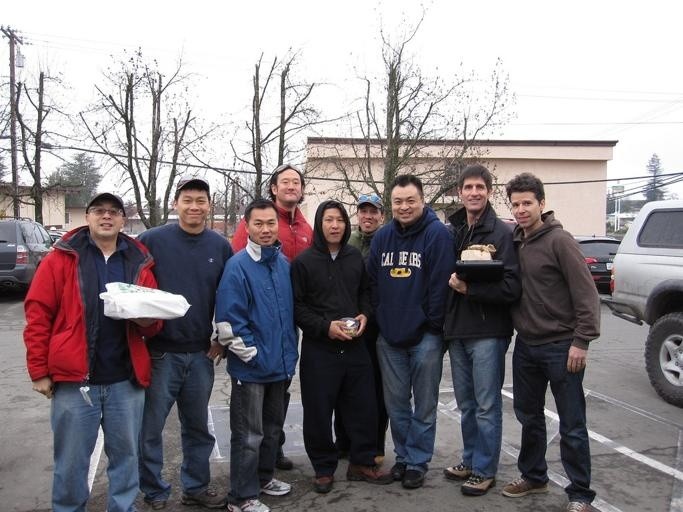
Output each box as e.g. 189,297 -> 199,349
573,233 -> 626,296
43,225 -> 69,245
498,216 -> 518,234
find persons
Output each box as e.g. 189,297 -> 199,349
214,199 -> 299,512
505,172 -> 600,512
23,193 -> 164,512
446,164 -> 522,495
334,194 -> 388,471
135,177 -> 235,511
291,201 -> 394,494
230,163 -> 314,469
367,173 -> 457,488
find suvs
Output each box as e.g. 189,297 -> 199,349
603,197 -> 682,407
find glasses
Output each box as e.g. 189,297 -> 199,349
87,208 -> 123,217
358,195 -> 380,202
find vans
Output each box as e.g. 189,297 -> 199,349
0,212 -> 58,295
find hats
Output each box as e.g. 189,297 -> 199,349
177,176 -> 210,191
456,247 -> 505,268
355,195 -> 384,209
86,193 -> 127,217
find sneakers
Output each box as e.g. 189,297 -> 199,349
566,500 -> 590,512
227,496 -> 273,512
151,500 -> 167,512
259,477 -> 290,496
502,475 -> 549,498
333,443 -> 426,489
275,457 -> 294,470
444,462 -> 496,496
181,487 -> 226,508
313,476 -> 334,493
